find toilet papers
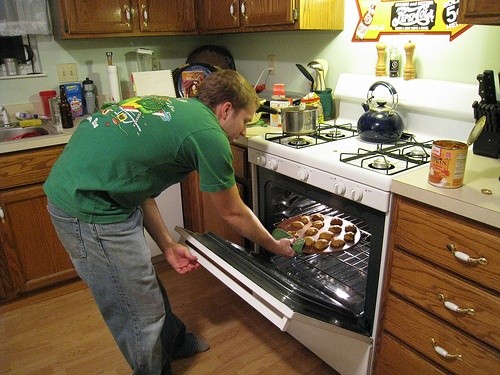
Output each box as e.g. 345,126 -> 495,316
107,66 -> 120,102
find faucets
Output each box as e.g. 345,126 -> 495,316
0,106 -> 11,127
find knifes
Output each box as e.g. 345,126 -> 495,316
477,70 -> 500,104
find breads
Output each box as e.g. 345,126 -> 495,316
287,215 -> 357,249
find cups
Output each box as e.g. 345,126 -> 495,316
39,90 -> 57,117
18,58 -> 27,75
310,87 -> 334,121
4,58 -> 18,75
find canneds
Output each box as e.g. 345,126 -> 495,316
428,115 -> 486,189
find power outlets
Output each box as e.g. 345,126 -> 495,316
57,64 -> 79,83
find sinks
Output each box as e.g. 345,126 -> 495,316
0,118 -> 64,143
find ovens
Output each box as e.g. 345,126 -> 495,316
173,163 -> 389,375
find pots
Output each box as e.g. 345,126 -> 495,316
255,99 -> 319,135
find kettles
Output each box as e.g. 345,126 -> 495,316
356,81 -> 404,144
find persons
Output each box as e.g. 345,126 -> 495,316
42,71 -> 301,375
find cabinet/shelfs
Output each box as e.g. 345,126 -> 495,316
181,145 -> 247,251
52,0 -> 345,40
0,145 -> 76,304
369,194 -> 500,375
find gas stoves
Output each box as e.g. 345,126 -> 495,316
247,73 -> 482,213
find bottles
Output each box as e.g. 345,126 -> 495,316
59,87 -> 73,128
390,48 -> 401,77
81,77 -> 96,115
